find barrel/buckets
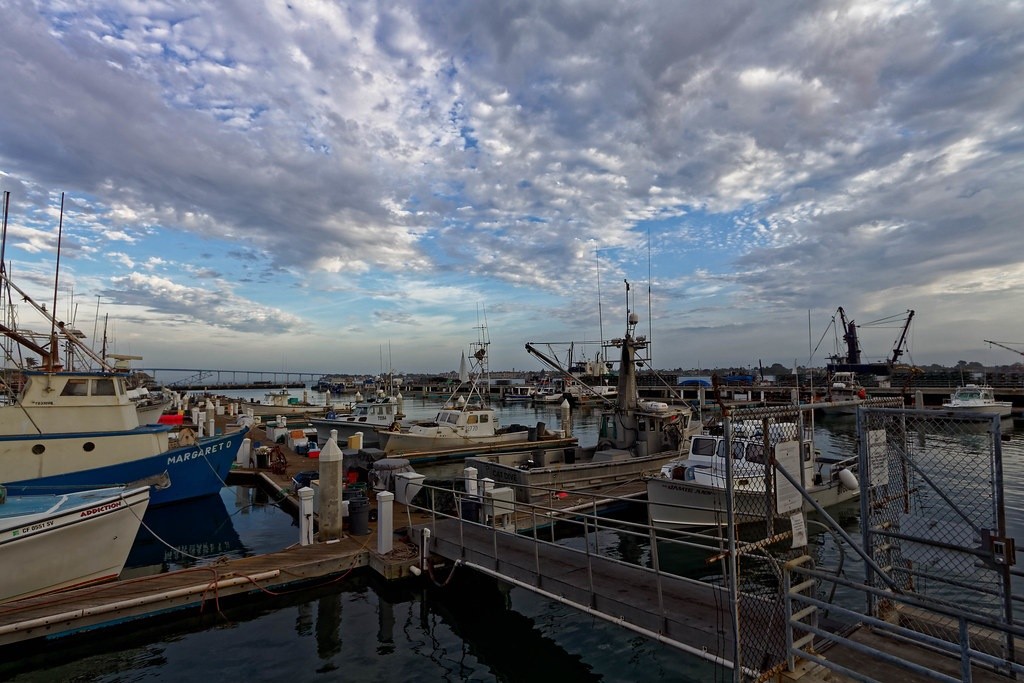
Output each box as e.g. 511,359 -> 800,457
348,482 -> 367,496
348,497 -> 371,535
343,488 -> 362,500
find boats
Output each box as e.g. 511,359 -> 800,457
0,189 -> 1014,604
